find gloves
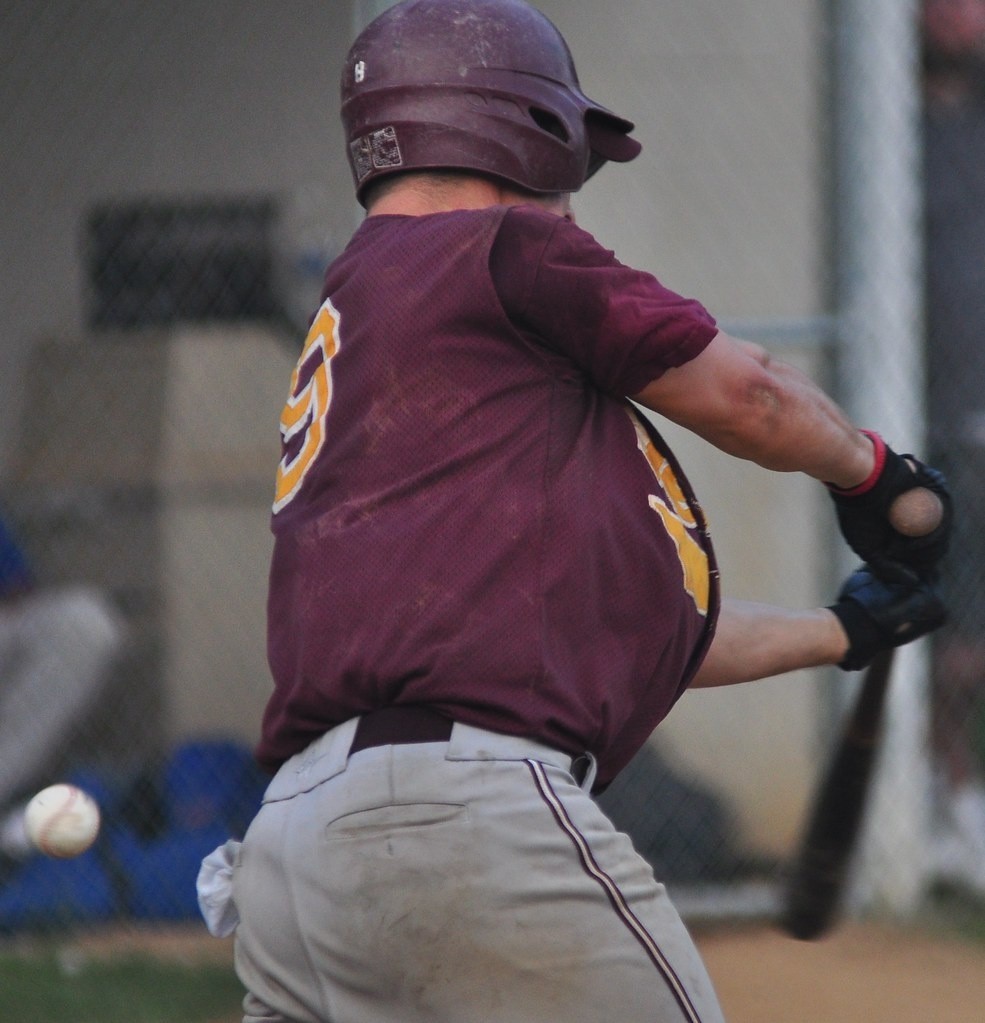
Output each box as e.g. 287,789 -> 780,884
821,429 -> 954,566
826,563 -> 948,671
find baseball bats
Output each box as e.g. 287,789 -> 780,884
771,484 -> 947,943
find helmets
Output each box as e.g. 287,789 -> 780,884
341,0 -> 642,209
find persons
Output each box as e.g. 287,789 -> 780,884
193,0 -> 956,1023
0,520 -> 133,822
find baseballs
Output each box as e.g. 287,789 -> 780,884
24,781 -> 103,863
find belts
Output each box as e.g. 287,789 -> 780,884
348,705 -> 589,787
